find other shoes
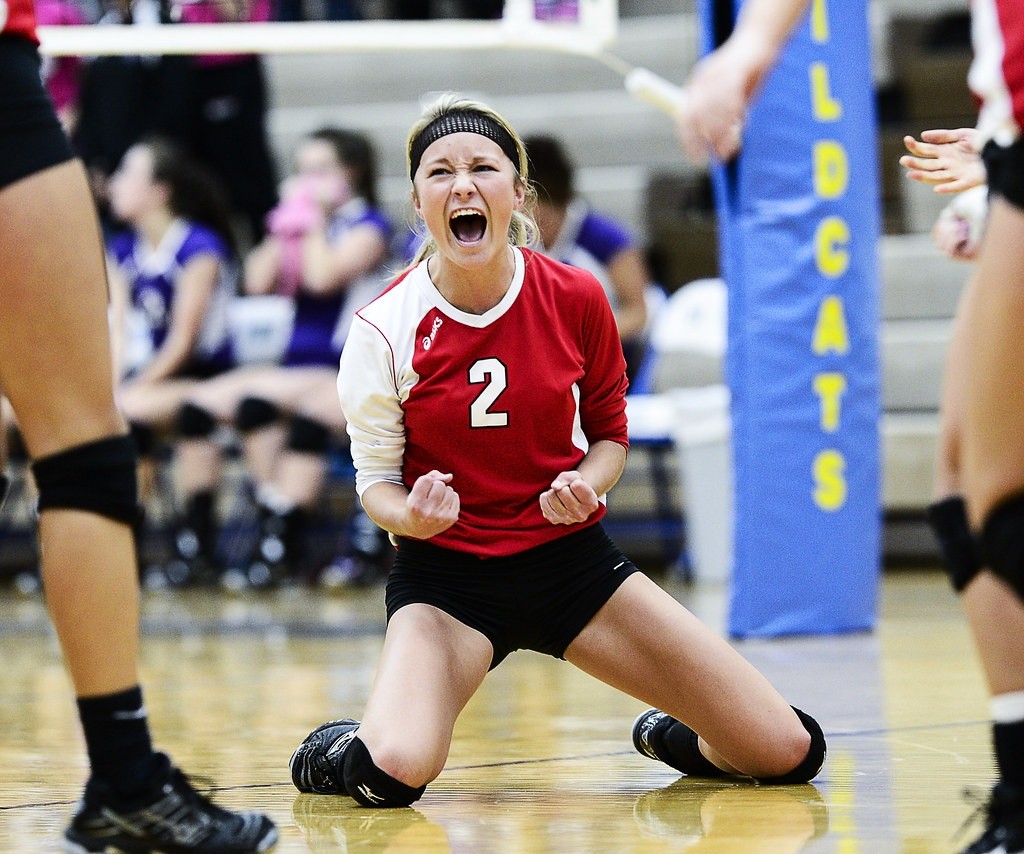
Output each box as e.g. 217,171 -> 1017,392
233,500 -> 318,590
158,487 -> 224,587
958,781 -> 1024,854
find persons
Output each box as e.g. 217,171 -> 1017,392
525,137 -> 647,395
222,128 -> 394,596
101,134 -> 238,584
289,100 -> 827,808
682,0 -> 1024,854
0,0 -> 277,854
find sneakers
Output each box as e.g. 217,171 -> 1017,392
630,707 -> 679,762
57,752 -> 281,854
289,719 -> 363,798
